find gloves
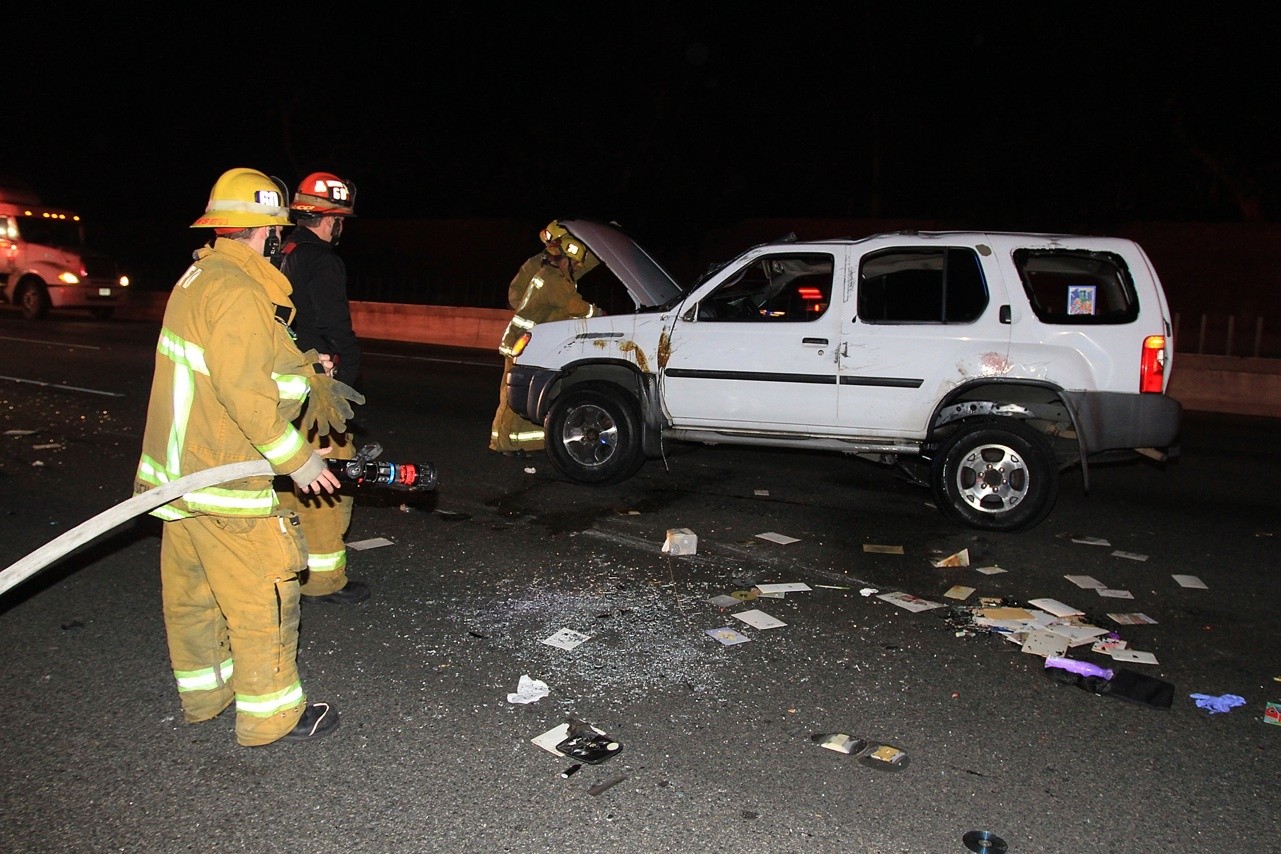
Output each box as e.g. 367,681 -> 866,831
304,349 -> 365,436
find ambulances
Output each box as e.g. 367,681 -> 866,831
0,188 -> 130,322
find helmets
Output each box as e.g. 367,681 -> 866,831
540,220 -> 568,244
189,167 -> 296,228
546,236 -> 588,261
290,172 -> 353,217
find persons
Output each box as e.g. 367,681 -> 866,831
275,170 -> 371,604
134,166 -> 339,744
491,219 -> 607,457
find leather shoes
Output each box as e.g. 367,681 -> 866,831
301,581 -> 371,604
280,703 -> 340,743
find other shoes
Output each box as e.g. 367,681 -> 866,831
535,449 -> 546,457
512,449 -> 536,458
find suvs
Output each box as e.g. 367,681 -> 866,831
504,220 -> 1184,535
